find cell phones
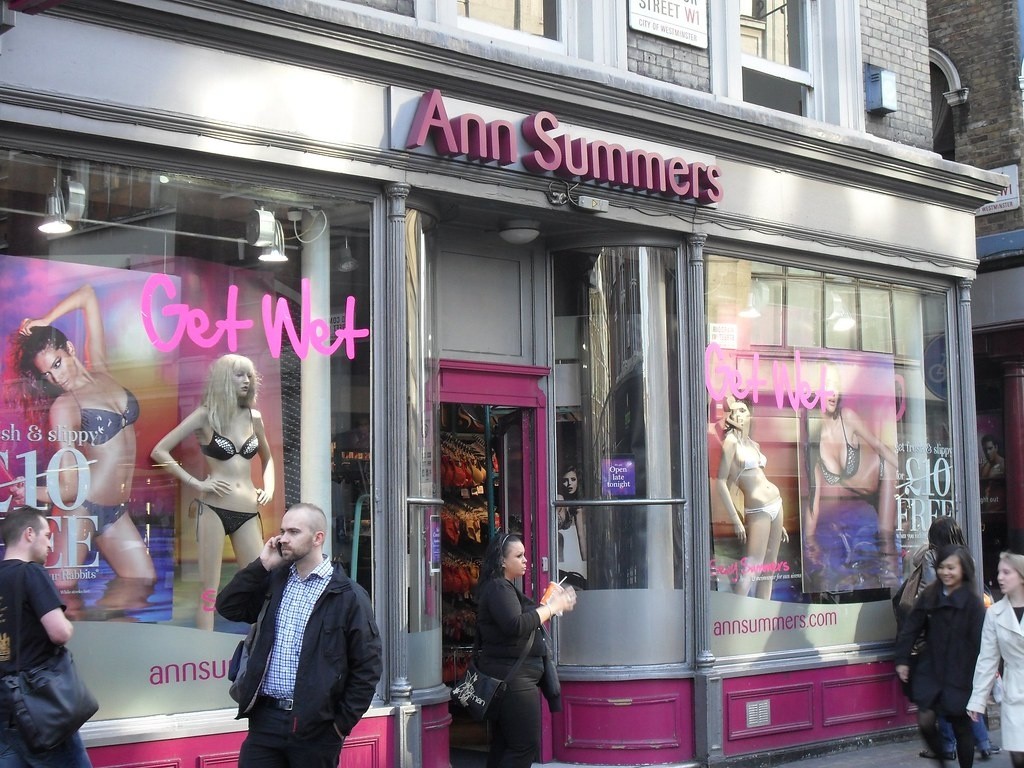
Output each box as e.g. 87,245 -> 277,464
276,540 -> 282,557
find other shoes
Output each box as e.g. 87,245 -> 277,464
990,744 -> 1002,754
983,751 -> 991,759
920,750 -> 956,760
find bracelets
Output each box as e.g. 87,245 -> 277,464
185,476 -> 192,488
548,605 -> 552,620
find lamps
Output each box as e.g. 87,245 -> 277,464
257,214 -> 289,263
36,178 -> 72,234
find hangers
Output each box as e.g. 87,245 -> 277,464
441,432 -> 502,663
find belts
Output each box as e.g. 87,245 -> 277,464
256,700 -> 294,711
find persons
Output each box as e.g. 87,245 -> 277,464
893,516 -> 1024,768
214,503 -> 383,768
557,466 -> 586,562
150,354 -> 276,632
981,435 -> 1004,476
804,369 -> 898,576
3,284 -> 157,586
0,506 -> 94,768
717,398 -> 789,600
475,533 -> 577,768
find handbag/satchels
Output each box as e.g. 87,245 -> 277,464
228,581 -> 273,703
0,644 -> 99,754
450,653 -> 510,723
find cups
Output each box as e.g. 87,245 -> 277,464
540,581 -> 565,606
